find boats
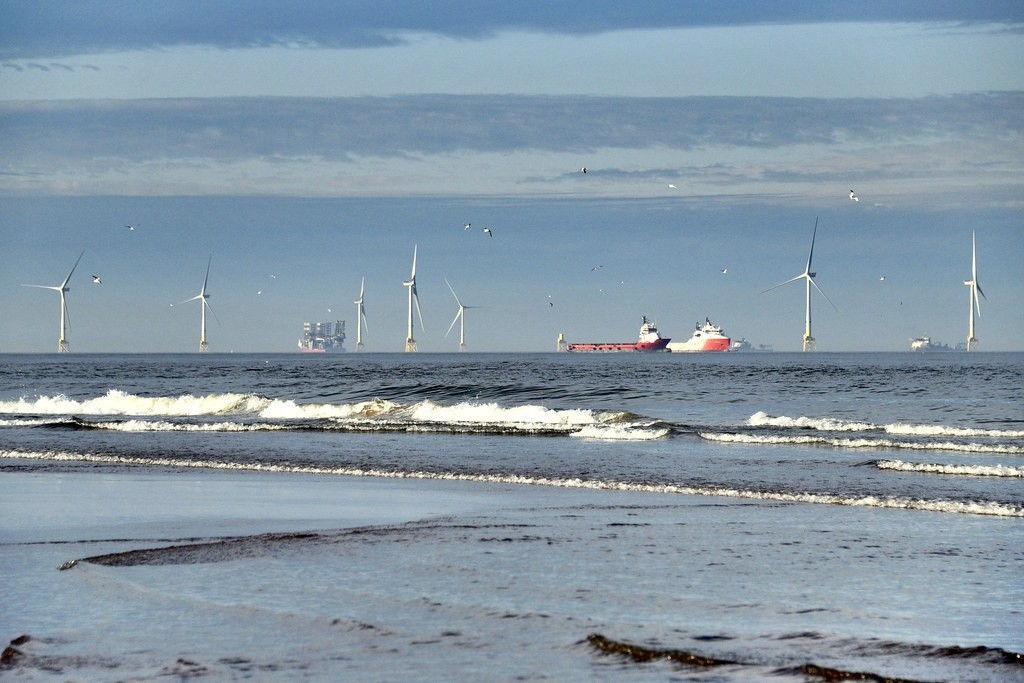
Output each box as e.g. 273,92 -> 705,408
911,332 -> 966,352
729,337 -> 774,354
566,316 -> 672,352
666,317 -> 731,352
298,320 -> 347,354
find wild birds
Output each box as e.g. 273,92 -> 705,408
463,223 -> 471,230
91,276 -> 102,284
849,190 -> 859,202
549,301 -> 554,307
580,168 -> 588,174
880,276 -> 885,280
123,224 -> 140,231
482,227 -> 493,239
668,184 -> 676,190
721,268 -> 728,273
591,265 -> 604,272
